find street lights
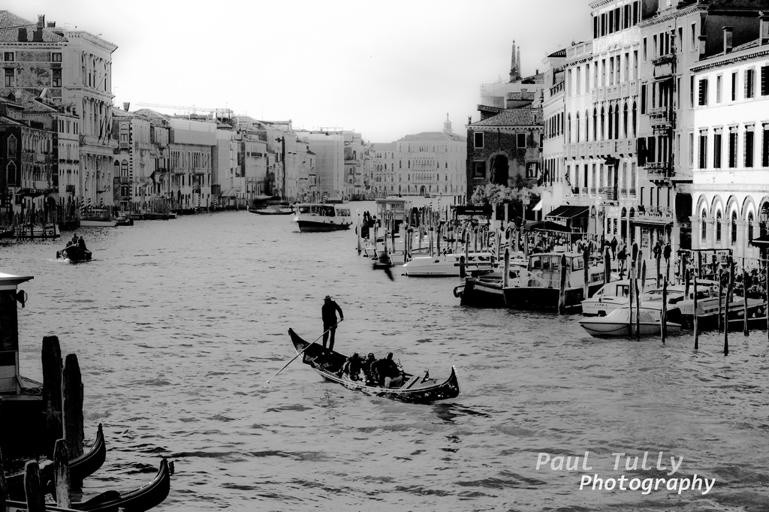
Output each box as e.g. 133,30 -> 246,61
759,208 -> 769,268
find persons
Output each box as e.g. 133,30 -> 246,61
588,234 -> 671,269
341,351 -> 405,388
67,233 -> 88,251
321,295 -> 344,356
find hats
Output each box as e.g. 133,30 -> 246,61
323,295 -> 333,300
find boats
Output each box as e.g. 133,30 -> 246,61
0,420 -> 111,497
295,202 -> 353,232
286,326 -> 461,403
0,268 -> 61,446
70,456 -> 176,512
19,205 -> 180,236
65,241 -> 93,259
250,199 -> 303,215
